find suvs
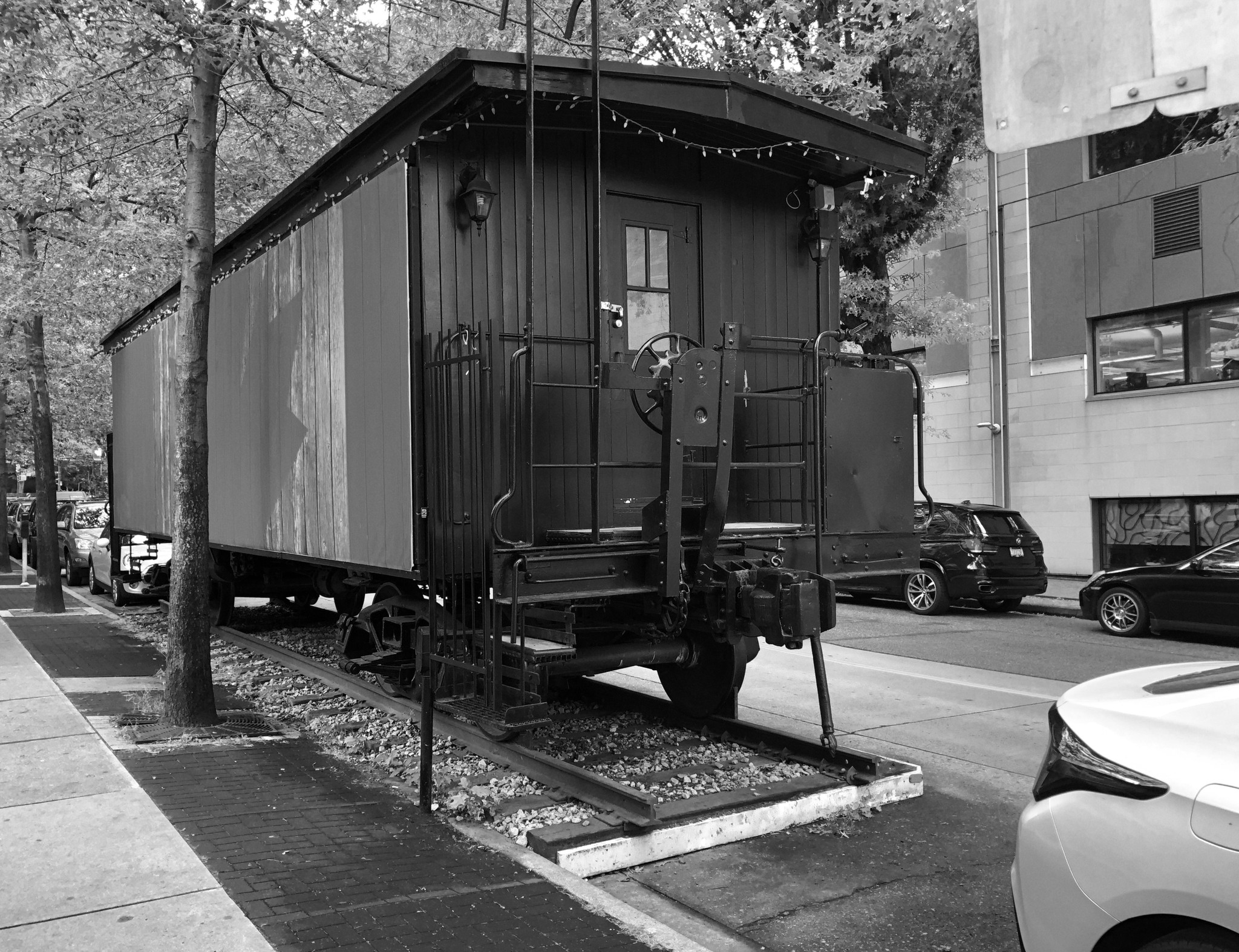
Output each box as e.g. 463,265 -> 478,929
844,500 -> 1050,615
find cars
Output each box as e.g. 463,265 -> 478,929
87,515 -> 175,606
1006,661 -> 1238,952
2,490 -> 112,586
1079,537 -> 1239,636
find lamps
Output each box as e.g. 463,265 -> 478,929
459,162 -> 499,238
802,217 -> 833,275
